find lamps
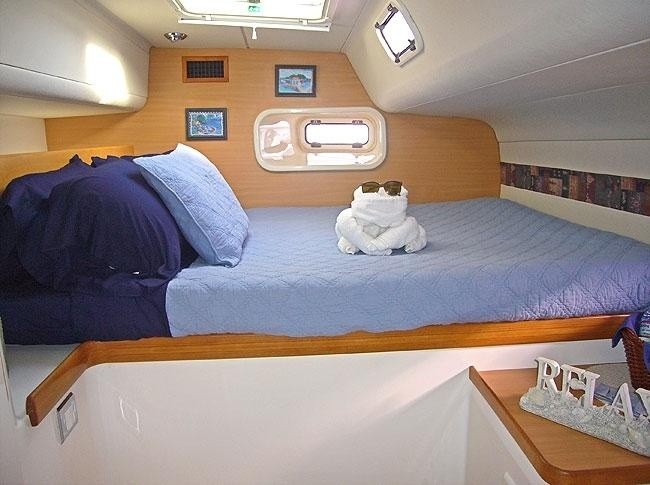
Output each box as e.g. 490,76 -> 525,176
251,27 -> 257,40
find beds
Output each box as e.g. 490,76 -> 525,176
1,143 -> 649,484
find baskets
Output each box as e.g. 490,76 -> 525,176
623,330 -> 650,389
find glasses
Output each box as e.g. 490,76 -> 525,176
358,181 -> 402,196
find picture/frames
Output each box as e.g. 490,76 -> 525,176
185,108 -> 228,141
275,64 -> 317,97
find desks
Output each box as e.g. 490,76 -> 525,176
466,363 -> 648,485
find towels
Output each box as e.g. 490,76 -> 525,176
334,182 -> 428,256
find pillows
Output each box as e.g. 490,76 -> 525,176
0,144 -> 250,298
